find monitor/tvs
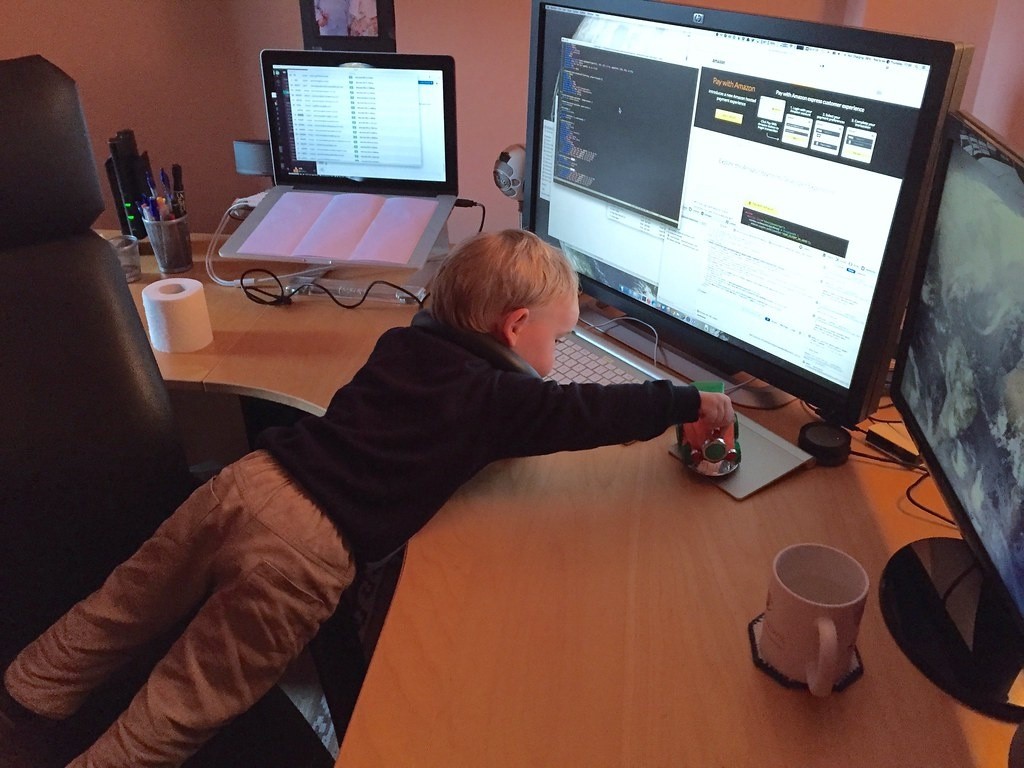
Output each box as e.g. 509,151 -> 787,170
522,0 -> 975,421
878,111 -> 1024,722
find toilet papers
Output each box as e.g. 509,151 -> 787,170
140,277 -> 214,353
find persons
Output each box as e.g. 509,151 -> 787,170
0,226 -> 735,768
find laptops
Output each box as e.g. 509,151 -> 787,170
217,48 -> 459,271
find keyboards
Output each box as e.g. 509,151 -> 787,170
541,323 -> 689,387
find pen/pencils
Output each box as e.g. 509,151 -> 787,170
135,163 -> 192,268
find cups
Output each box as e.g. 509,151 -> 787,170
106,235 -> 140,282
758,544 -> 869,696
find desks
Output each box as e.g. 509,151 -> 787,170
92,229 -> 1024,768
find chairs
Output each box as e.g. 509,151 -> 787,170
0,55 -> 336,768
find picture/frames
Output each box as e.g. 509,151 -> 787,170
300,0 -> 397,53
